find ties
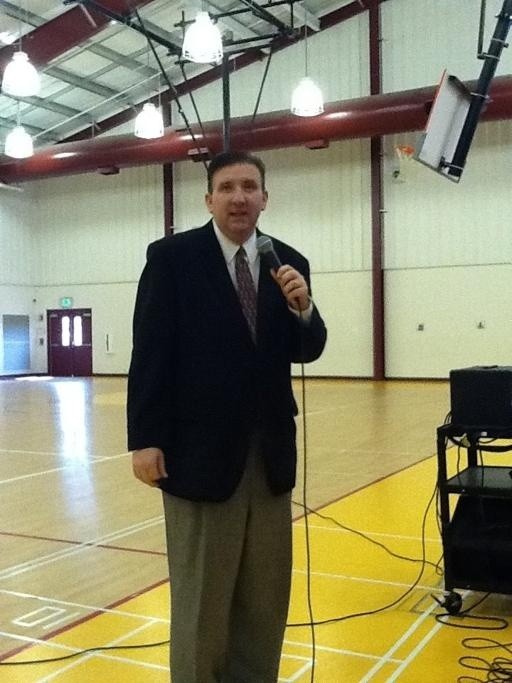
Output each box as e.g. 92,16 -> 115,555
235,246 -> 256,338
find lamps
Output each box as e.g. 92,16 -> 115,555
176,1 -> 230,69
2,98 -> 39,159
132,33 -> 172,142
286,1 -> 324,117
1,1 -> 43,96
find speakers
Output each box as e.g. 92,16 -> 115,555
450,366 -> 512,427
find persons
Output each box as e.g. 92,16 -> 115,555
125,148 -> 327,683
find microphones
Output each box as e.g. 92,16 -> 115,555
255,236 -> 299,301
